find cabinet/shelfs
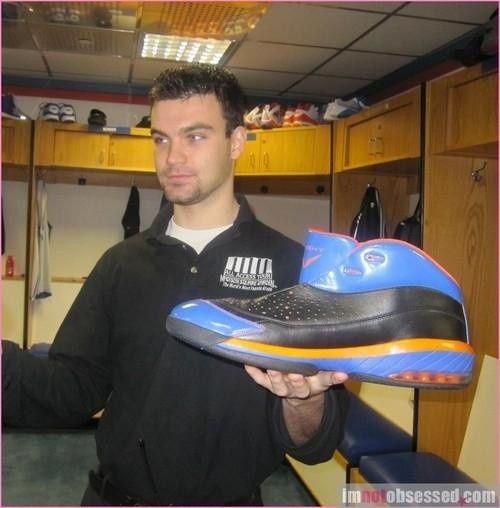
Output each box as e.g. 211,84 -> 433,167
2,56 -> 499,176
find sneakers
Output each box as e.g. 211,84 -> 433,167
165,226 -> 474,390
135,115 -> 151,128
1,94 -> 32,121
38,102 -> 76,122
87,109 -> 108,127
242,96 -> 371,130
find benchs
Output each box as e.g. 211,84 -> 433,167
284,385 -> 500,506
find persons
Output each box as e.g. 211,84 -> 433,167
0,62 -> 350,507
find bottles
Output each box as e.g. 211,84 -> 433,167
6,256 -> 14,276
